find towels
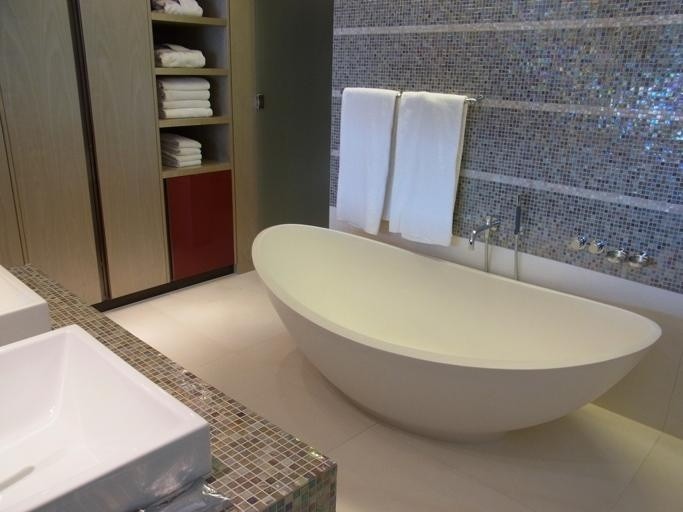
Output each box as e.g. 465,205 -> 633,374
333,86 -> 471,247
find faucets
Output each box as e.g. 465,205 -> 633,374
469,225 -> 492,247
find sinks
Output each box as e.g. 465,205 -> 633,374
0,324 -> 210,511
0,265 -> 49,345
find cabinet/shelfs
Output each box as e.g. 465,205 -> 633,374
1,2 -> 170,305
148,2 -> 234,280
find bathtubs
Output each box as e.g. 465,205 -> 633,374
251,223 -> 662,437
